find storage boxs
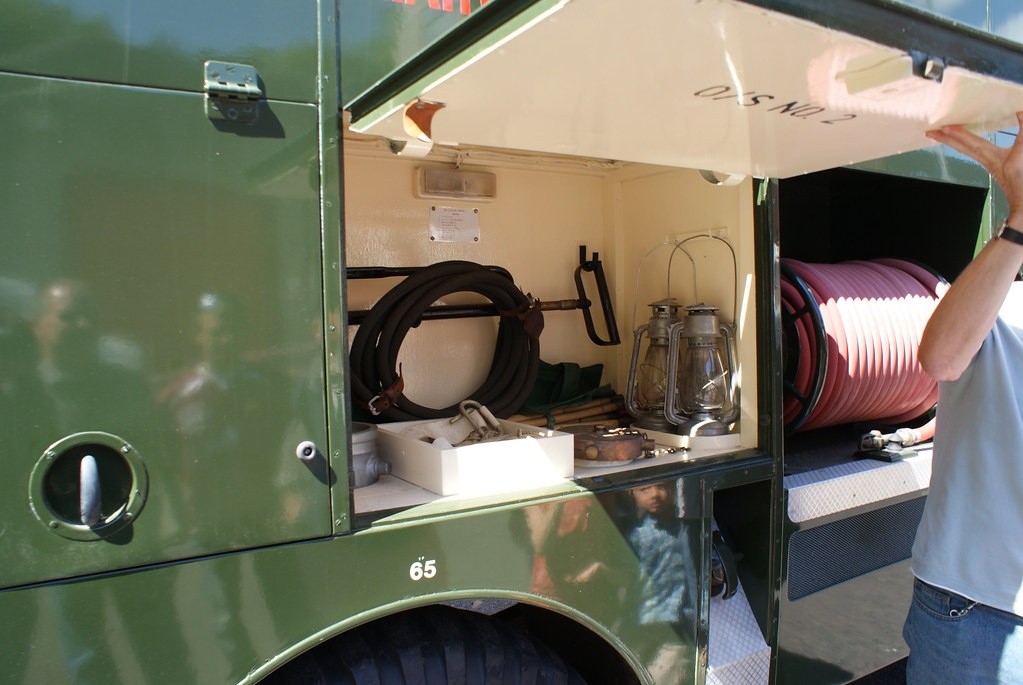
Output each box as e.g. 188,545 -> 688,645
376,417 -> 574,497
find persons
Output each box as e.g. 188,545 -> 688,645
507,480 -> 704,685
901,111 -> 1023,685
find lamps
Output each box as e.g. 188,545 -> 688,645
665,234 -> 740,437
624,240 -> 699,435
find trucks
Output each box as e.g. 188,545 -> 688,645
0,0 -> 1023,685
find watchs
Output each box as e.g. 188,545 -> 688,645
994,215 -> 1023,248
